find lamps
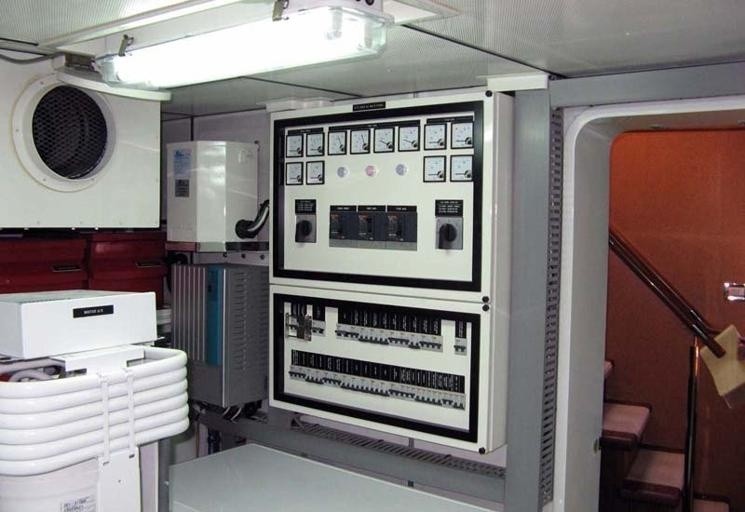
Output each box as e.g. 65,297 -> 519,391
90,0 -> 394,89
